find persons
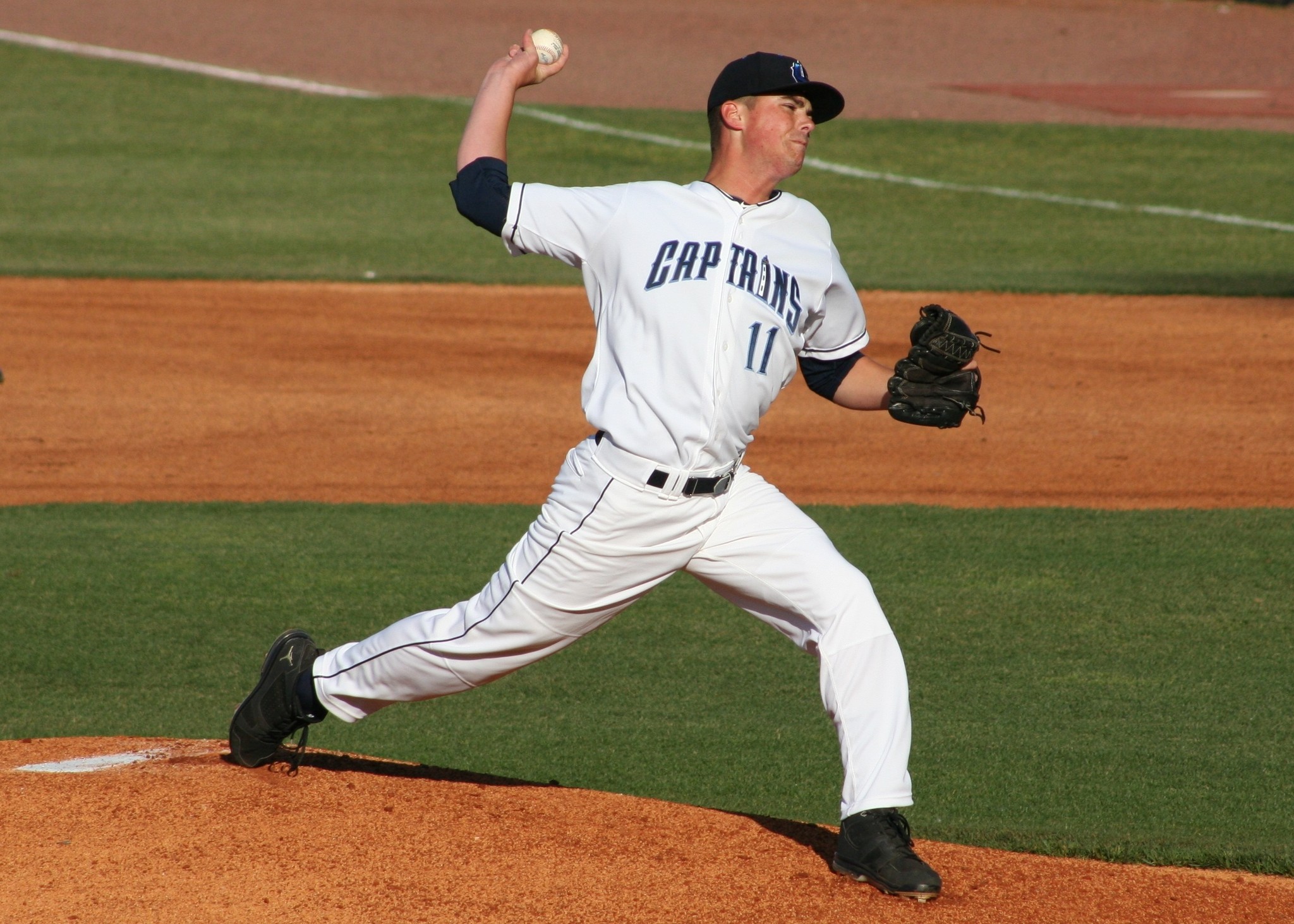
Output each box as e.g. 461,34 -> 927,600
228,30 -> 982,900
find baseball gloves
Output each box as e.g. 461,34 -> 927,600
885,304 -> 984,429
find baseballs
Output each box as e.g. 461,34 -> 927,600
530,28 -> 564,66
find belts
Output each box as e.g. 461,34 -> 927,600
595,430 -> 734,496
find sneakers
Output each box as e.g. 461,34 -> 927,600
228,629 -> 327,771
832,808 -> 942,902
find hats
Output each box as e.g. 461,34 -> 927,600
710,52 -> 845,124
